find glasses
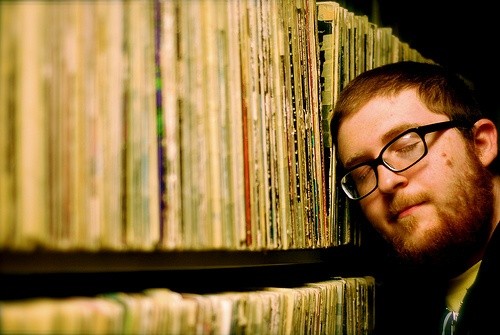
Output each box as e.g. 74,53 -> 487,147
339,117 -> 477,202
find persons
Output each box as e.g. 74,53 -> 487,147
330,59 -> 499,335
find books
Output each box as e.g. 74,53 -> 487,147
0,0 -> 435,335
